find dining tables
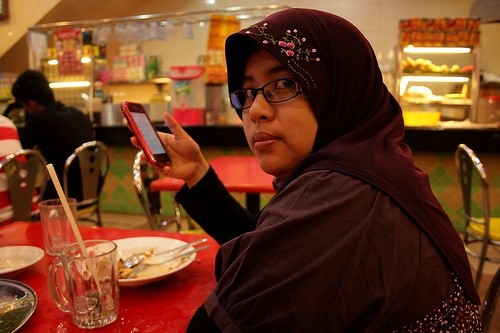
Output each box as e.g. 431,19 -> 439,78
0,220 -> 221,333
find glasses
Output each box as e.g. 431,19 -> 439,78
230,78 -> 306,110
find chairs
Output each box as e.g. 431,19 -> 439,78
455,144 -> 500,298
0,141 -> 182,234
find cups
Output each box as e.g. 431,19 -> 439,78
38,198 -> 77,256
47,238 -> 120,328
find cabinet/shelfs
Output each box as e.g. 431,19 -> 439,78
391,43 -> 480,122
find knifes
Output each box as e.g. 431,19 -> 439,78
125,244 -> 211,280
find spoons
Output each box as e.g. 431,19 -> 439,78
123,237 -> 208,267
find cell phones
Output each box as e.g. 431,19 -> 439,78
120,101 -> 171,167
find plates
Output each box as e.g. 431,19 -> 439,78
0,246 -> 45,277
0,278 -> 37,333
83,237 -> 197,288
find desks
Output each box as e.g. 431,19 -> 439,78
207,156 -> 278,206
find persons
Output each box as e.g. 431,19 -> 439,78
11,69 -> 104,223
0,114 -> 42,230
130,8 -> 481,333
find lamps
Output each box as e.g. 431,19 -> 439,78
81,26 -> 93,63
46,31 -> 58,64
